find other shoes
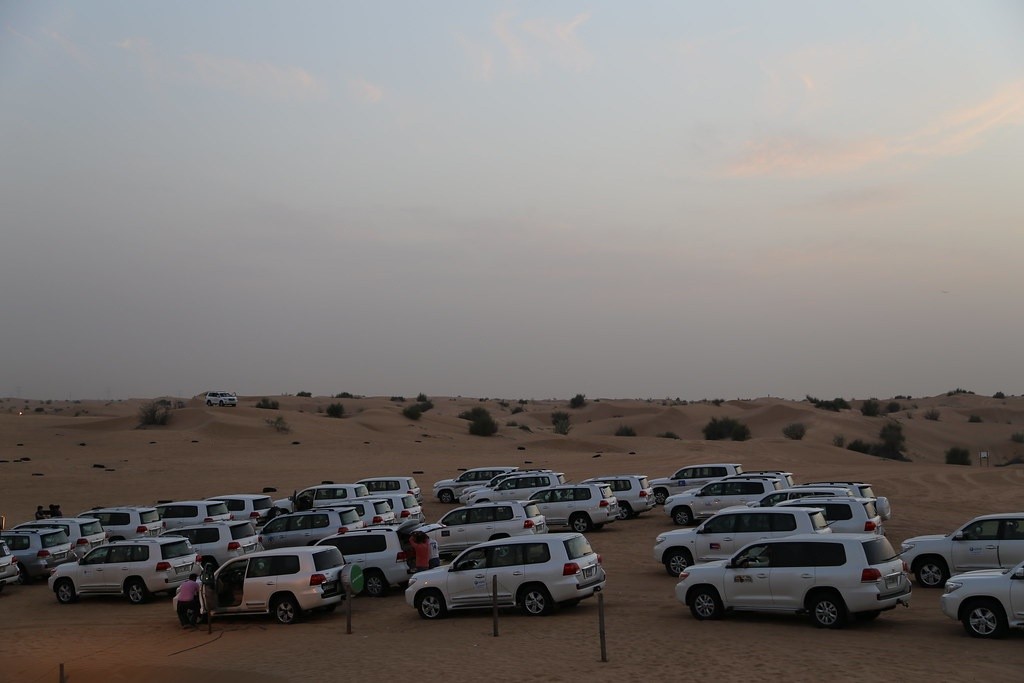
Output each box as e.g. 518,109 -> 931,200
178,623 -> 190,630
190,622 -> 200,630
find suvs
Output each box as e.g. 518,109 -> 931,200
522,483 -> 623,534
405,531 -> 607,617
459,467 -> 553,506
172,546 -> 364,626
0,476 -> 428,606
206,391 -> 238,407
648,463 -> 744,505
432,466 -> 519,505
941,559 -> 1024,638
899,513 -> 1024,589
767,494 -> 891,537
675,532 -> 913,629
313,518 -> 446,598
433,499 -> 550,562
720,469 -> 795,490
564,475 -> 657,520
716,480 -> 876,515
465,472 -> 568,509
653,501 -> 832,578
663,474 -> 784,527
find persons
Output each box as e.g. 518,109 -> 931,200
176,573 -> 201,629
409,530 -> 440,572
35,504 -> 62,520
